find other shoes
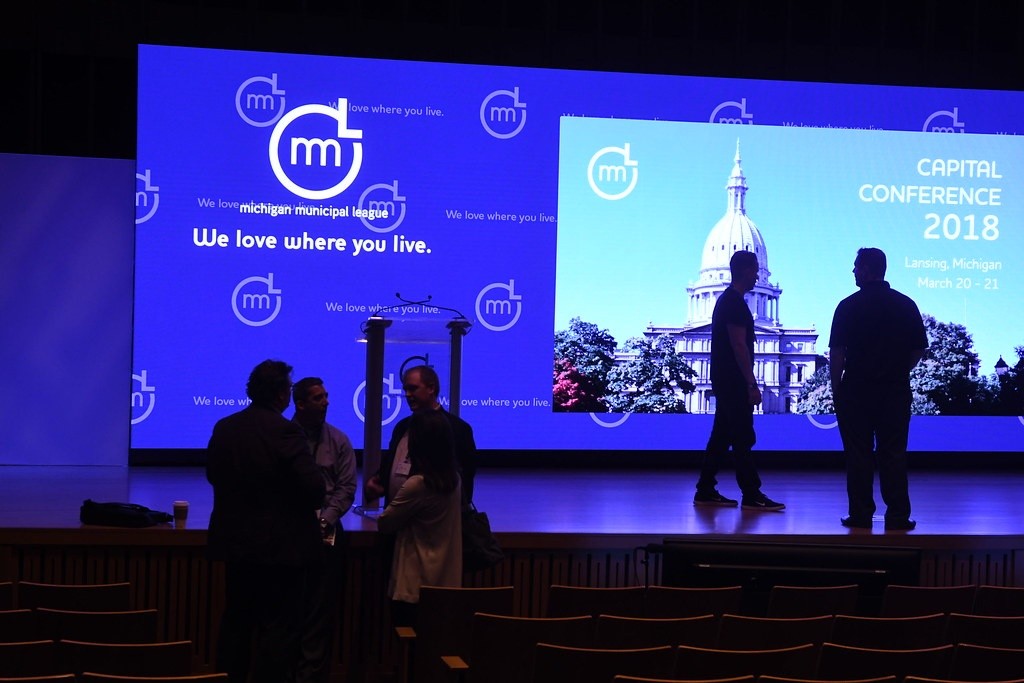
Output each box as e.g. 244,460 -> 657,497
840,515 -> 873,529
884,519 -> 916,531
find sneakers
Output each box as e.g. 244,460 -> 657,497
742,494 -> 786,511
693,490 -> 738,507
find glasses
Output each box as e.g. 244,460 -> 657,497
286,381 -> 296,392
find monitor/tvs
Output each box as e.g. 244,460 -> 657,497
659,538 -> 921,606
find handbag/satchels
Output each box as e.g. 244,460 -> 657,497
461,485 -> 506,570
79,498 -> 174,528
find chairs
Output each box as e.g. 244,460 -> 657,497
393,584 -> 1024,683
0,580 -> 229,683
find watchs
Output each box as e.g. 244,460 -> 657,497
747,380 -> 759,389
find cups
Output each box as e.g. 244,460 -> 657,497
172,500 -> 190,529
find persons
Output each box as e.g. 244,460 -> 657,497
202,360 -> 336,682
691,250 -> 787,512
287,363 -> 480,627
827,248 -> 929,532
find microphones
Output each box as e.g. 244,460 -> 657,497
372,292 -> 466,319
